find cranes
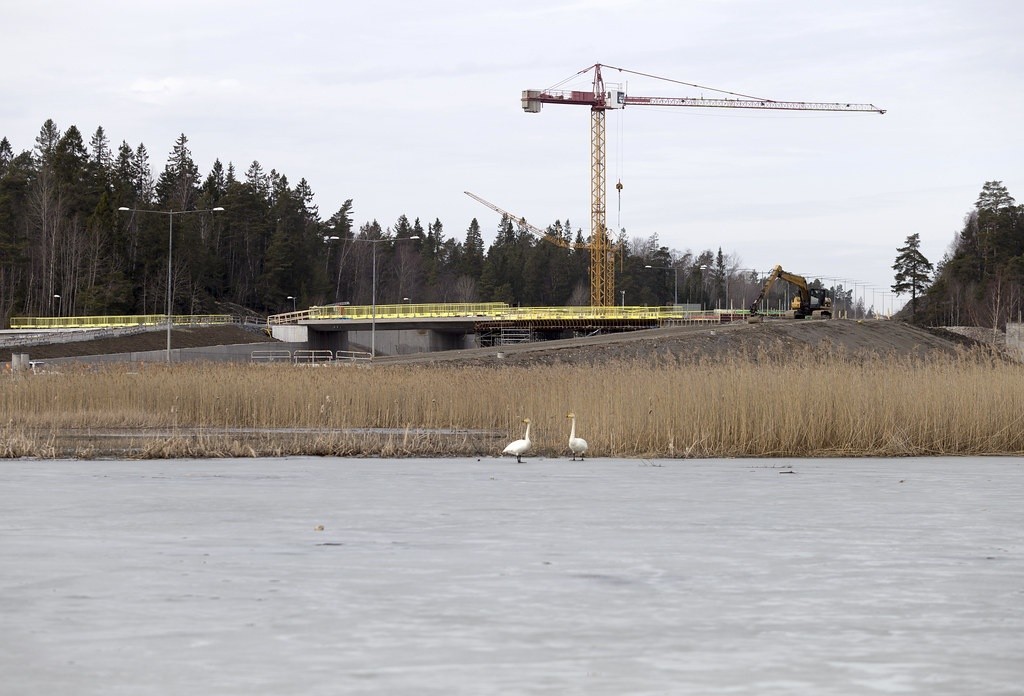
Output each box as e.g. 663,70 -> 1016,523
522,63 -> 888,307
464,191 -> 625,308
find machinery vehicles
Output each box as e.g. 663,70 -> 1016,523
747,265 -> 833,324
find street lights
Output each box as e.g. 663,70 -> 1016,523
645,265 -> 678,304
329,235 -> 420,359
118,206 -> 224,365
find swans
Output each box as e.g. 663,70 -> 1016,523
501,418 -> 532,464
566,413 -> 588,461
